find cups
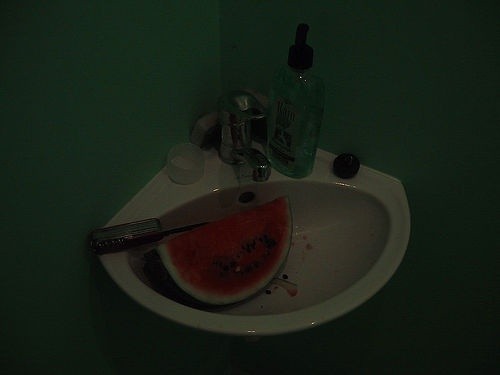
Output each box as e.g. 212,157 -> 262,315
167,143 -> 204,186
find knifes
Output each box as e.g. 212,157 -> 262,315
89,218 -> 215,257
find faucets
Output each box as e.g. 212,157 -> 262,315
215,90 -> 272,181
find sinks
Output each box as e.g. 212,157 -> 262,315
93,116 -> 411,341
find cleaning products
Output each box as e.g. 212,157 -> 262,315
265,23 -> 325,179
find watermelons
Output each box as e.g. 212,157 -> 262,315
143,194 -> 293,306
